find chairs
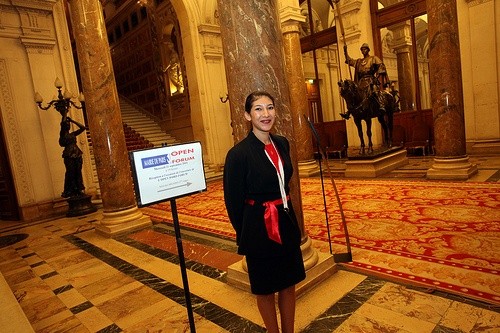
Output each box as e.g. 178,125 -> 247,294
314,131 -> 346,159
401,124 -> 431,158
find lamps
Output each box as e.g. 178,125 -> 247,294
30,76 -> 89,114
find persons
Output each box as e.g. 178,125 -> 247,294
223,91 -> 306,333
338,44 -> 389,117
58,117 -> 87,199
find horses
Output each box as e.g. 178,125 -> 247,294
337,79 -> 396,155
